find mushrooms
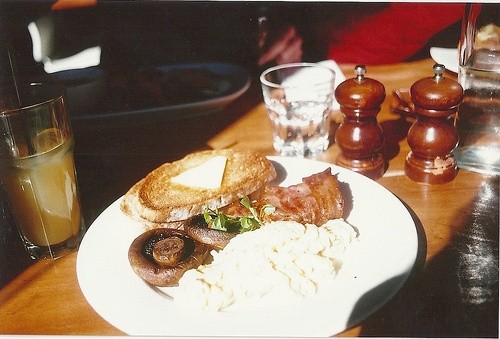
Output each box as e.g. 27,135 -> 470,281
184,213 -> 240,249
128,228 -> 207,287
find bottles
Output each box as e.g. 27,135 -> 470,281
452,3 -> 500,174
335,65 -> 385,179
405,64 -> 463,184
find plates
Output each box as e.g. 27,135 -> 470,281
69,62 -> 251,123
431,46 -> 460,77
78,154 -> 420,337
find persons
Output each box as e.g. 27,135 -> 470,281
256,2 -> 484,80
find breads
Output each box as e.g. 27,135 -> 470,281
120,178 -> 187,229
135,148 -> 277,223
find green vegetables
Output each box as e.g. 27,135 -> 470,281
203,195 -> 274,234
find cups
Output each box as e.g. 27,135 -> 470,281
1,80 -> 87,261
260,63 -> 336,160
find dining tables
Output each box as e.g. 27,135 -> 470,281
0,57 -> 499,338
